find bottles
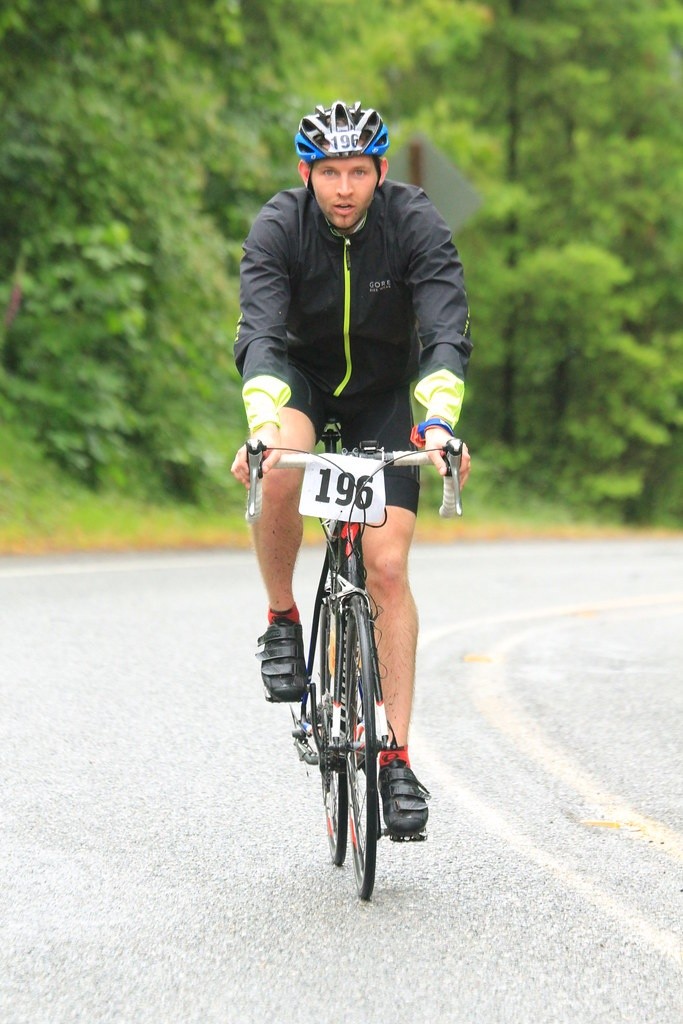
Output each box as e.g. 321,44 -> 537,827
328,614 -> 338,677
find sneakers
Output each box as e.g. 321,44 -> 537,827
254,618 -> 307,703
361,754 -> 431,836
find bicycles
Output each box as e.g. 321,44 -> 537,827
246,436 -> 462,903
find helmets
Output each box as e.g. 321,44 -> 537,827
294,101 -> 390,164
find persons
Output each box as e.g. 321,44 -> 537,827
231,102 -> 472,836
